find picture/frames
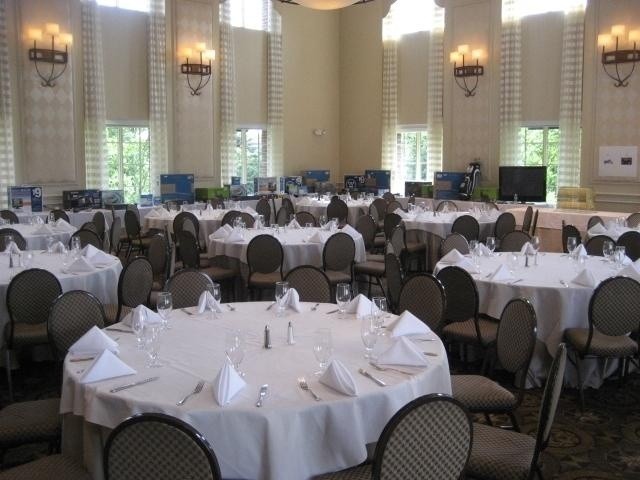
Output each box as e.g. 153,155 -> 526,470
598,146 -> 637,176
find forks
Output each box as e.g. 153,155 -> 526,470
301,380 -> 320,400
369,361 -> 413,376
177,383 -> 204,406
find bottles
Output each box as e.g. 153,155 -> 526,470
263,321 -> 296,349
514,194 -> 518,205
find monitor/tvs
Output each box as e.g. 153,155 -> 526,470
498,166 -> 547,205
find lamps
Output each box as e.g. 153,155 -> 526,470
176,43 -> 216,96
598,25 -> 640,87
29,23 -> 73,87
278,0 -> 375,10
450,45 -> 489,96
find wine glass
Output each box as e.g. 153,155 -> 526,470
469,236 -> 625,275
131,282 -> 387,376
0,213 -> 55,234
308,191 -> 374,204
5,235 -> 82,274
408,201 -> 490,220
233,214 -> 338,240
162,198 -> 241,219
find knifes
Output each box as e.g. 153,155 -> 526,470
257,385 -> 268,406
110,378 -> 159,392
